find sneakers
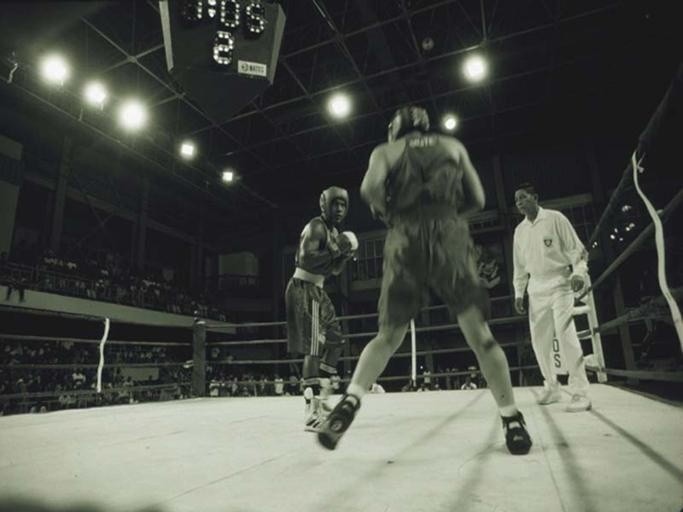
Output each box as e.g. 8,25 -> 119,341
567,395 -> 592,413
320,395 -> 362,451
303,403 -> 333,433
502,413 -> 532,456
537,383 -> 564,406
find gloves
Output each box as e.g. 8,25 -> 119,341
327,232 -> 359,258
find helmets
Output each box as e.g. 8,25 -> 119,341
319,187 -> 350,223
388,109 -> 428,140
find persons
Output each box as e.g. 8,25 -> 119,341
507,182 -> 595,412
330,357 -> 487,393
283,184 -> 360,430
0,238 -> 306,419
313,106 -> 535,455
